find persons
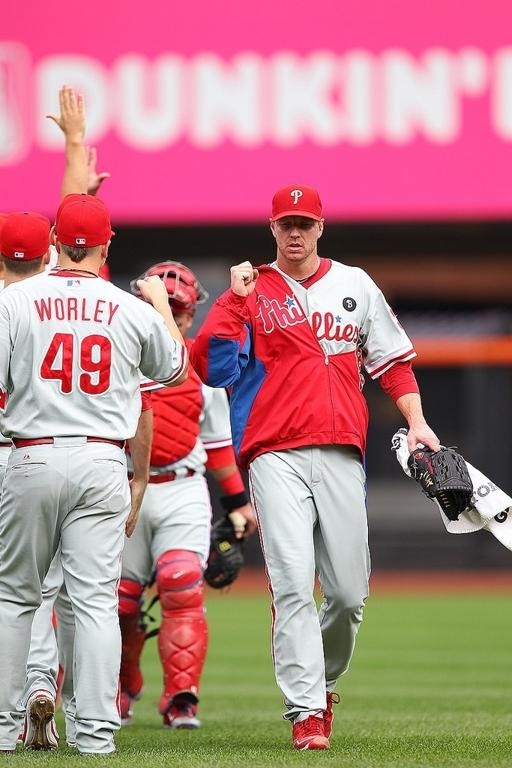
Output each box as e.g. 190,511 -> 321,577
190,179 -> 444,752
2,83 -> 260,762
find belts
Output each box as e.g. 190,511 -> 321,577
128,469 -> 195,483
14,437 -> 124,449
0,442 -> 11,448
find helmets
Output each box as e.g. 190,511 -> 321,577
130,261 -> 209,314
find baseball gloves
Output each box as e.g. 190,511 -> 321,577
408,447 -> 472,519
204,516 -> 246,588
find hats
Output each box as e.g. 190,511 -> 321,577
0,211 -> 51,261
271,184 -> 322,224
57,192 -> 115,247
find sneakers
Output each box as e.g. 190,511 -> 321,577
120,692 -> 133,724
24,691 -> 60,751
0,743 -> 17,751
293,693 -> 334,750
164,695 -> 201,729
